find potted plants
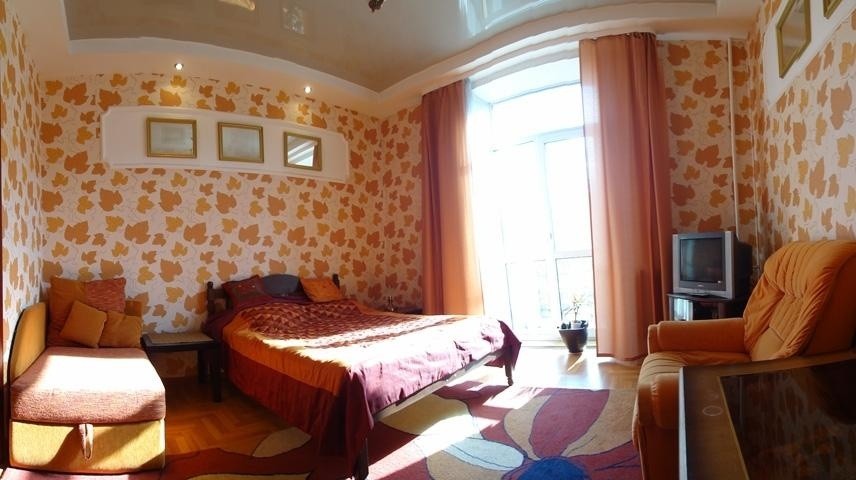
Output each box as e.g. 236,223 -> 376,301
558,295 -> 589,353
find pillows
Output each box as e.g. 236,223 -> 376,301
49,277 -> 128,344
59,302 -> 107,348
222,275 -> 274,313
126,296 -> 146,316
301,276 -> 348,303
101,309 -> 144,352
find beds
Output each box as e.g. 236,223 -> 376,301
206,273 -> 520,479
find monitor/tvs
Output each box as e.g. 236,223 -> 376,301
674,231 -> 737,299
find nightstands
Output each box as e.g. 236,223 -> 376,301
142,332 -> 221,405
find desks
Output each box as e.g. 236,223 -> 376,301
667,292 -> 750,321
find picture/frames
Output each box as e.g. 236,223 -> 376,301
284,131 -> 323,171
145,116 -> 198,160
775,1 -> 814,78
821,0 -> 844,19
218,121 -> 265,164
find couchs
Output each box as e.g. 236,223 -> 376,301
10,302 -> 167,475
631,242 -> 856,480
